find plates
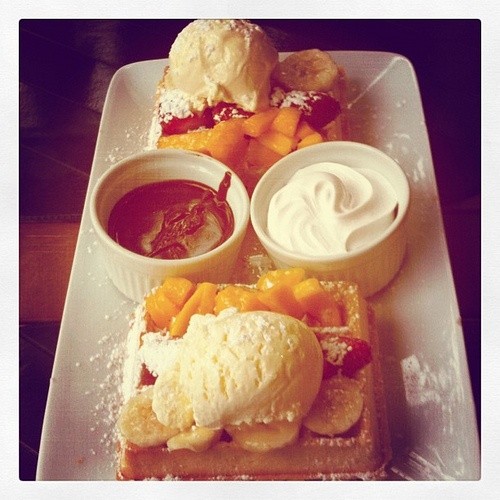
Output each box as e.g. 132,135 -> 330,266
35,49 -> 482,482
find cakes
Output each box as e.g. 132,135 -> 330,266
147,19 -> 351,195
115,269 -> 393,481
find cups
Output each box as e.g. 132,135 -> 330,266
90,148 -> 251,304
248,140 -> 410,298
